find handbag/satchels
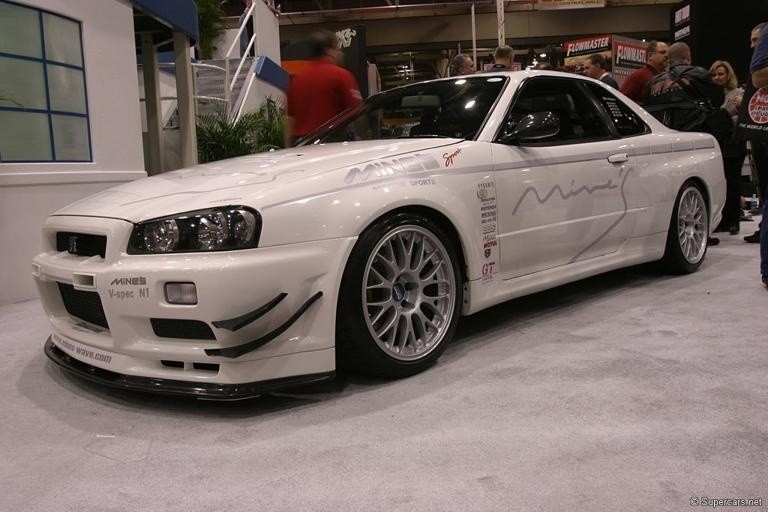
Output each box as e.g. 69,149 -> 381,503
707,108 -> 734,140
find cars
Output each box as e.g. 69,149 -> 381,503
31,69 -> 728,402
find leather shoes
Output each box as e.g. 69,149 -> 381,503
744,235 -> 760,243
707,238 -> 719,245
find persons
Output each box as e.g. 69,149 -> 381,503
621,41 -> 734,150
709,60 -> 748,234
744,24 -> 767,289
583,53 -> 618,90
284,28 -> 366,148
451,54 -> 476,75
479,45 -> 515,72
537,62 -> 574,118
